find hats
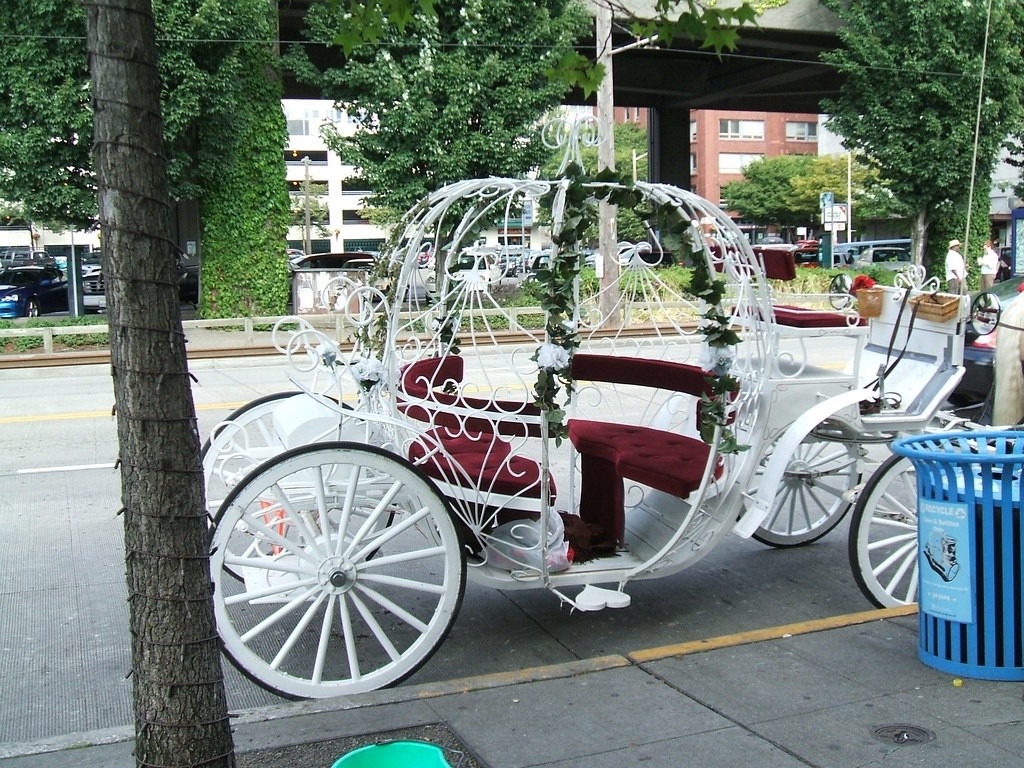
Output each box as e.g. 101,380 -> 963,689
948,239 -> 962,248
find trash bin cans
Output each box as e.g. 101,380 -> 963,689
890,430 -> 1024,681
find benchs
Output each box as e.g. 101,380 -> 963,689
566,353 -> 741,506
710,245 -> 871,381
396,356 -> 557,520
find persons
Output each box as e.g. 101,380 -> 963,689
978,241 -> 998,291
945,240 -> 969,294
993,282 -> 1024,428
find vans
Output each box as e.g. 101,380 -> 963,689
0,250 -> 50,267
287,252 -> 379,297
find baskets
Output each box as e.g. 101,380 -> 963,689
856,288 -> 883,317
908,293 -> 960,323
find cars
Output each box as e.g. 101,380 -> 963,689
994,247 -> 1013,282
424,253 -> 502,303
0,267 -> 69,319
341,260 -> 410,302
287,249 -> 304,259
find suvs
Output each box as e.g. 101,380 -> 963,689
81,264 -> 198,311
498,249 -> 553,276
743,240 -> 912,281
